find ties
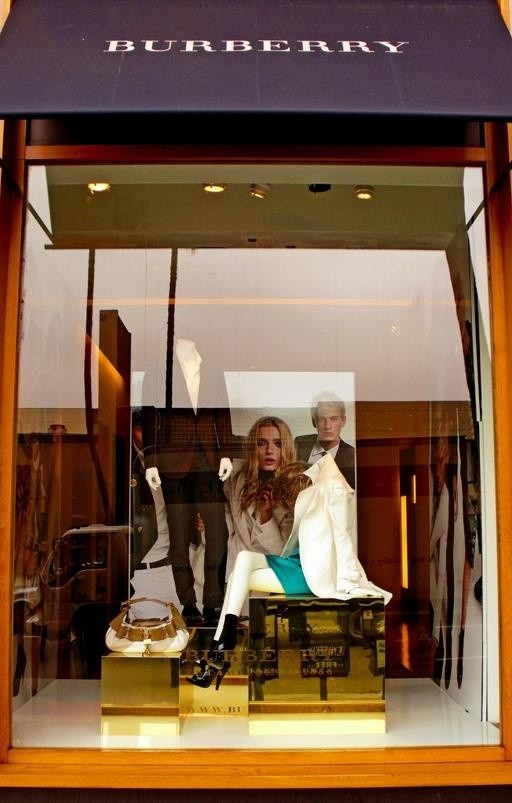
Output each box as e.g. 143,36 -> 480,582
322,452 -> 327,456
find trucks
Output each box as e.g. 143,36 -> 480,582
26,496 -> 427,661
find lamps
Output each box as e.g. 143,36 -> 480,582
354,185 -> 375,200
250,182 -> 272,199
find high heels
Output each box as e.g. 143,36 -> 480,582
185,641 -> 231,690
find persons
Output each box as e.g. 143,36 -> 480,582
124,402 -> 187,622
186,450 -> 395,692
295,392 -> 354,486
189,416 -> 303,571
140,337 -> 235,625
